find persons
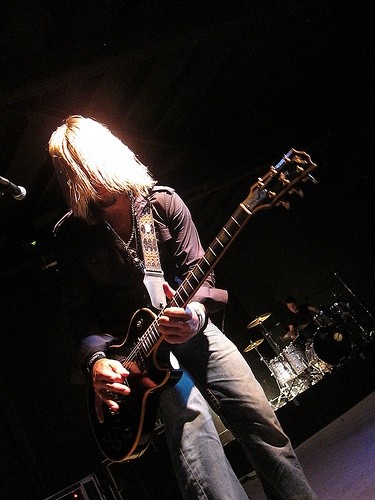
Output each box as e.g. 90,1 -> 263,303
49,115 -> 318,500
286,295 -> 320,339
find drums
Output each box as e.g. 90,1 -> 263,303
304,323 -> 354,373
268,342 -> 310,385
313,298 -> 350,330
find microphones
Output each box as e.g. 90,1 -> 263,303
0,176 -> 27,201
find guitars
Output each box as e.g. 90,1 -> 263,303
86,147 -> 319,463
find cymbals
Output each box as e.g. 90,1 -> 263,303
247,312 -> 271,329
243,338 -> 264,352
282,322 -> 308,339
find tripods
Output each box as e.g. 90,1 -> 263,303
244,322 -> 331,410
